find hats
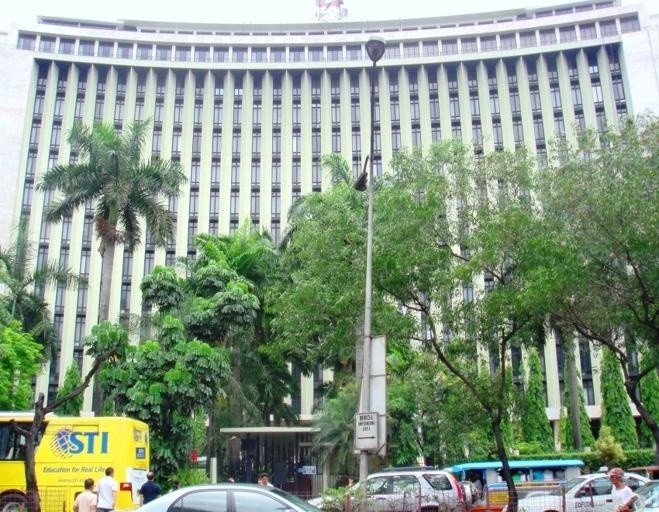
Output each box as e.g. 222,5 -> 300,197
607,468 -> 624,478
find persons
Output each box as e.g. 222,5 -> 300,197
469,474 -> 482,501
258,472 -> 273,488
509,470 -> 528,481
607,467 -> 636,512
72,479 -> 97,512
533,467 -> 564,480
168,480 -> 181,495
335,475 -> 353,490
138,471 -> 160,505
227,477 -> 235,483
95,467 -> 117,512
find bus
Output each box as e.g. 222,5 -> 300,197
1,412 -> 151,512
428,460 -> 586,512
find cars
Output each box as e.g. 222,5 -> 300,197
136,483 -> 325,512
504,465 -> 658,511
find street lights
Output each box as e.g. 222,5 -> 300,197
360,39 -> 386,512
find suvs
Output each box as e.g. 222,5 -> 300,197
307,468 -> 479,512
